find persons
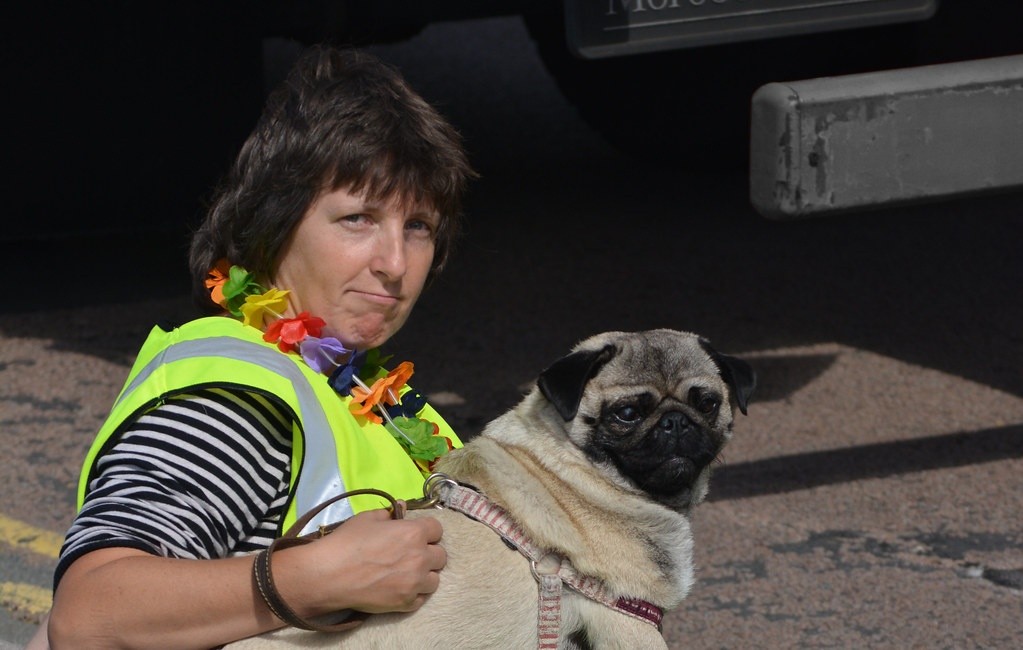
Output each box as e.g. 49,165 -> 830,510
47,48 -> 464,650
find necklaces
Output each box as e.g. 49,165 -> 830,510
205,259 -> 454,471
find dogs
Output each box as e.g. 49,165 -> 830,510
215,328 -> 751,649
